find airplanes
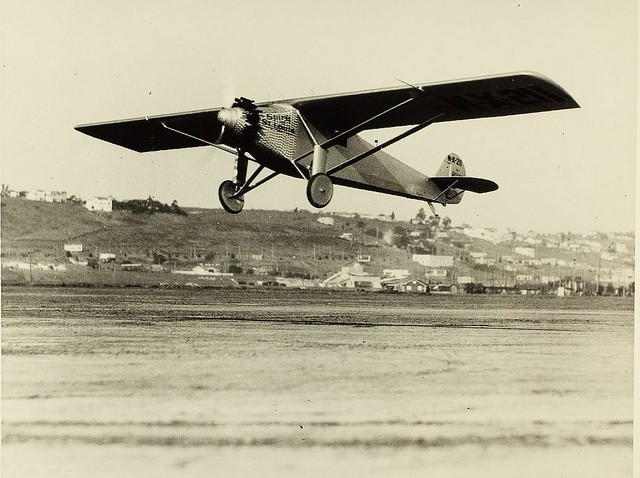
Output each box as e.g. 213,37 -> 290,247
74,72 -> 580,213
